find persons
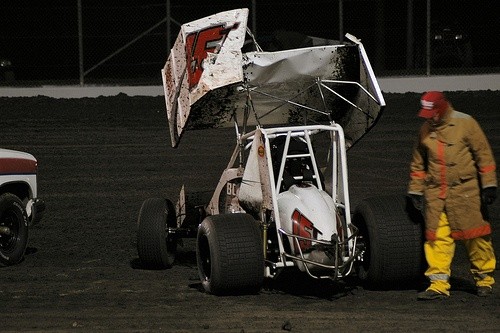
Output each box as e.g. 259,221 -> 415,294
406,91 -> 498,300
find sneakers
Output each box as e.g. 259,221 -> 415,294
478,286 -> 492,296
416,290 -> 447,300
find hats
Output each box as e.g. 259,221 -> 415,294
418,91 -> 446,118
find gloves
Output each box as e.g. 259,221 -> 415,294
482,187 -> 496,205
411,194 -> 425,210
